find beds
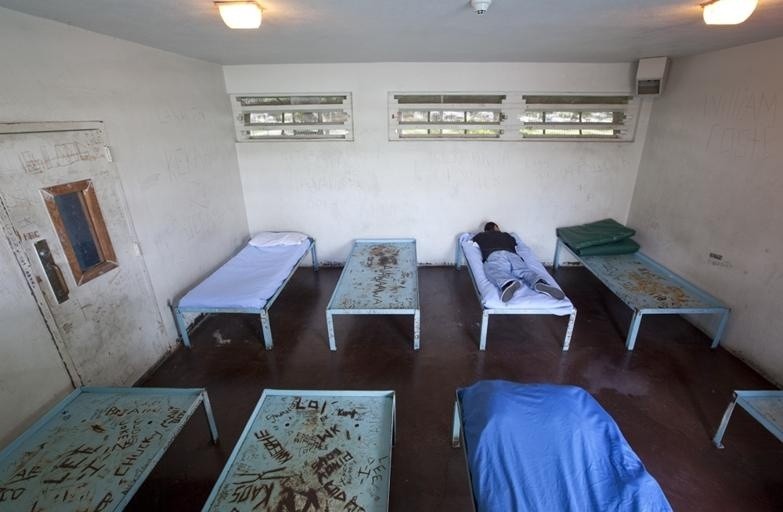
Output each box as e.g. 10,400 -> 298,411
553,236 -> 732,352
0,385 -> 219,512
325,237 -> 421,352
200,388 -> 397,512
712,389 -> 783,449
172,231 -> 317,352
456,230 -> 578,352
450,379 -> 674,512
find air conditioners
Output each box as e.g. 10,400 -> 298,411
635,57 -> 670,97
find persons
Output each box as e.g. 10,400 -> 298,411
471,221 -> 566,302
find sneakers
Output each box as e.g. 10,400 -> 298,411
501,279 -> 521,303
535,282 -> 564,300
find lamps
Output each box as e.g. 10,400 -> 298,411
214,1 -> 263,30
700,0 -> 759,26
